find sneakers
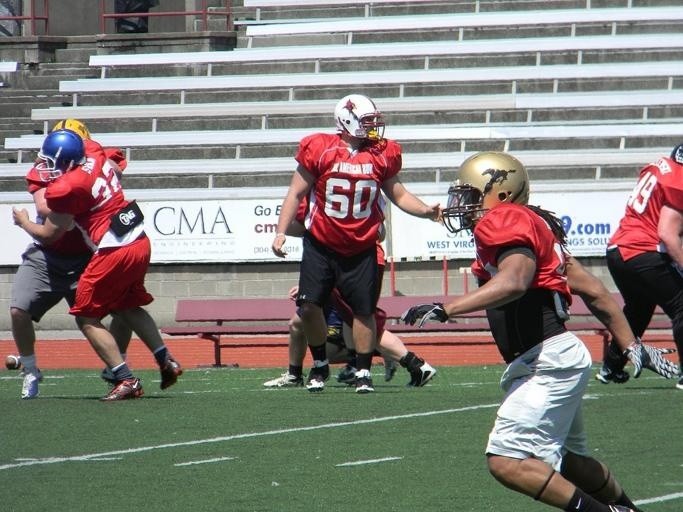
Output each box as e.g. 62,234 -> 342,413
262,372 -> 304,388
384,358 -> 397,382
334,364 -> 359,383
405,359 -> 437,387
19,367 -> 43,399
593,366 -> 630,383
160,357 -> 183,388
100,363 -> 119,384
355,368 -> 375,392
306,363 -> 329,391
98,377 -> 144,400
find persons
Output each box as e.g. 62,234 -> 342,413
596,144 -> 683,391
10,117 -> 136,400
12,128 -> 183,400
261,93 -> 443,392
399,150 -> 681,512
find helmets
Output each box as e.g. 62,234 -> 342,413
35,129 -> 85,181
670,143 -> 683,164
441,150 -> 530,232
53,119 -> 90,140
334,92 -> 385,141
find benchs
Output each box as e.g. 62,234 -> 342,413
160,294 -> 674,367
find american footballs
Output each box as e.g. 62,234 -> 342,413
5,355 -> 22,369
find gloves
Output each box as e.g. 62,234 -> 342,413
625,340 -> 680,381
399,299 -> 447,330
